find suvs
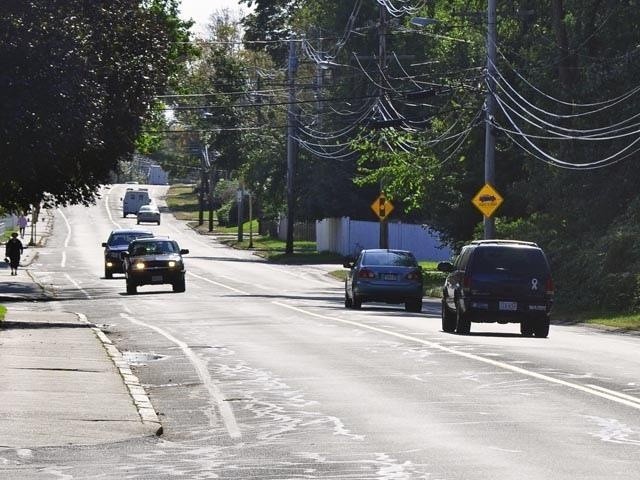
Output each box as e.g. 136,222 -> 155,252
437,239 -> 554,338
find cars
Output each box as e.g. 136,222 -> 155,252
341,247 -> 425,313
137,204 -> 161,225
101,229 -> 189,294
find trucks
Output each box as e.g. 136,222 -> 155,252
120,188 -> 151,218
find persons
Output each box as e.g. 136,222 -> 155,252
6,233 -> 23,275
16,214 -> 27,239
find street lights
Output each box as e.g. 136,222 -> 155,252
408,16 -> 499,240
201,112 -> 254,248
